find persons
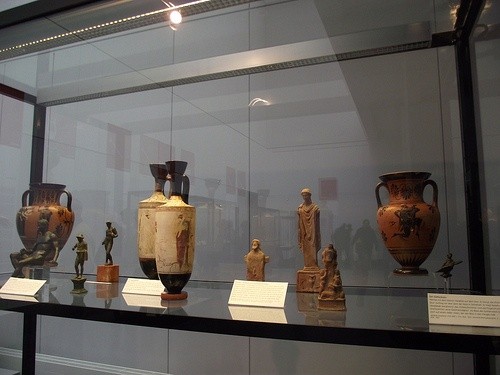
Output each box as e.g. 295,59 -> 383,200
10,219 -> 60,278
242,238 -> 269,281
102,221 -> 118,265
317,243 -> 345,299
72,233 -> 88,279
296,188 -> 321,270
